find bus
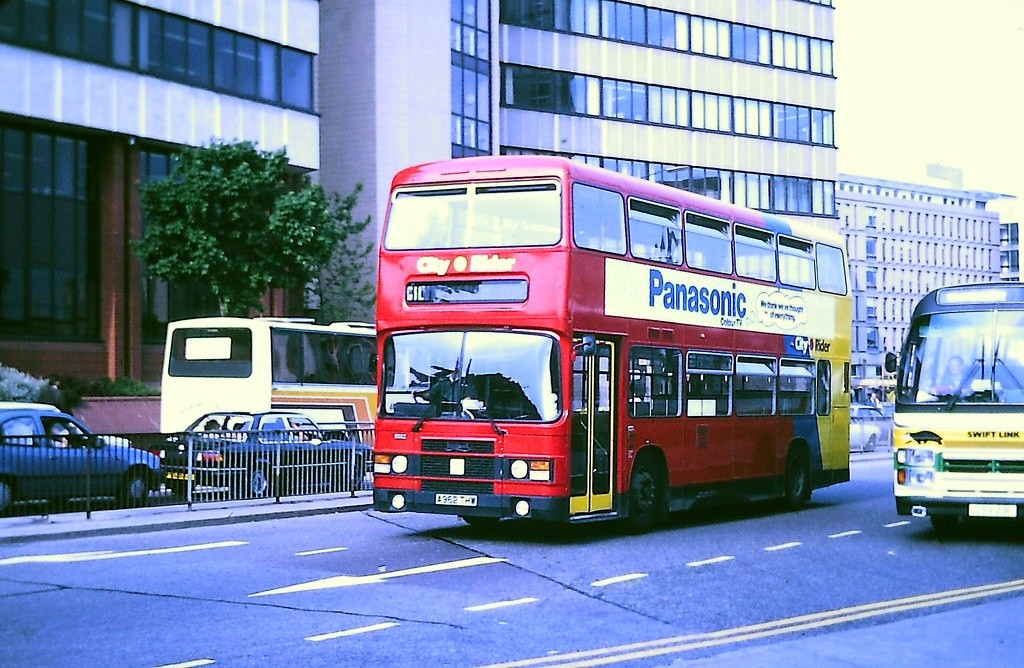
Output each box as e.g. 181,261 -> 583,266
158,314 -> 381,463
886,280 -> 1023,546
365,155 -> 854,527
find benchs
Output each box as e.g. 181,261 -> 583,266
631,387 -> 830,417
936,363 -> 1024,393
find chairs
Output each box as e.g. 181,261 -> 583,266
204,420 -> 283,441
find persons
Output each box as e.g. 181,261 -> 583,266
942,357 -> 972,394
288,418 -> 309,442
44,421 -> 68,448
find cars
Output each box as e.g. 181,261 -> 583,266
0,402 -> 163,513
153,406 -> 376,498
841,404 -> 891,449
